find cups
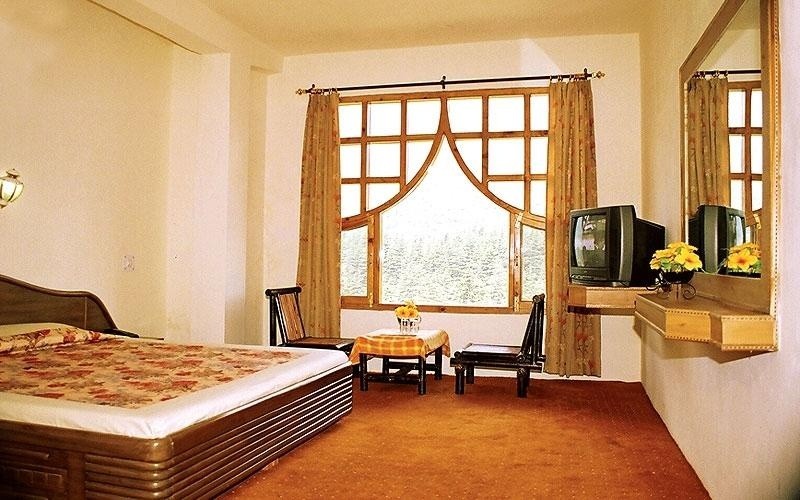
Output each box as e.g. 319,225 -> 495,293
400,318 -> 409,336
410,318 -> 418,336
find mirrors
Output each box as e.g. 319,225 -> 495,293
676,0 -> 782,317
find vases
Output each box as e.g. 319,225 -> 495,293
397,316 -> 420,335
646,269 -> 698,300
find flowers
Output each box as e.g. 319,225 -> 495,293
713,238 -> 762,275
649,240 -> 705,273
394,298 -> 420,320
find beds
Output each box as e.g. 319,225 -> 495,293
0,273 -> 355,499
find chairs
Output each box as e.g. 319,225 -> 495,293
449,292 -> 547,398
264,286 -> 359,379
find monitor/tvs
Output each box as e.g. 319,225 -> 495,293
688,205 -> 746,275
569,205 -> 665,287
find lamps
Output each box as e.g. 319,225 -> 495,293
0,165 -> 25,210
